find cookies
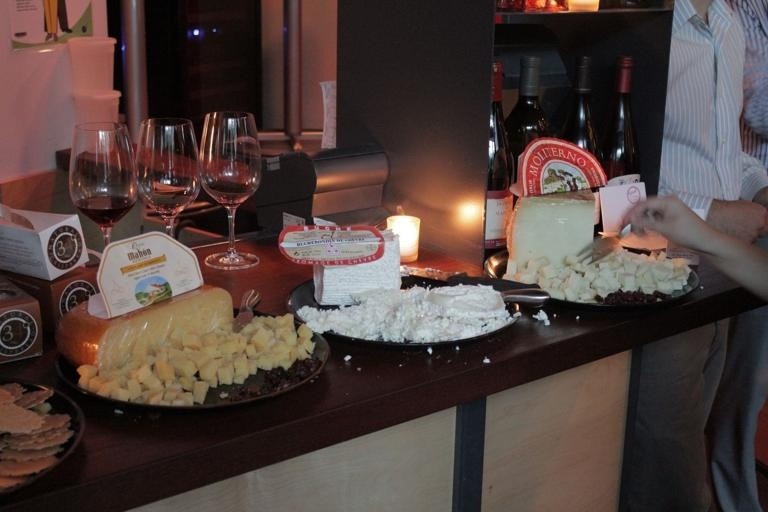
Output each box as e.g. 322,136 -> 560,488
0,383 -> 75,492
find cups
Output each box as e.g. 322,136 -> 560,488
495,0 -> 599,13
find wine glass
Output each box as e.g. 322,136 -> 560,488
196,110 -> 261,272
68,122 -> 137,246
134,116 -> 201,241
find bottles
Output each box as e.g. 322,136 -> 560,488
501,55 -> 553,194
483,61 -> 515,254
596,55 -> 638,182
553,52 -> 604,174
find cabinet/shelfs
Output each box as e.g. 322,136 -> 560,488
336,7 -> 676,277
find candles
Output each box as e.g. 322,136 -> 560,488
384,215 -> 421,264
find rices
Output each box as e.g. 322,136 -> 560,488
295,282 -> 550,364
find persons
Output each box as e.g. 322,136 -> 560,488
621,0 -> 768,512
704,0 -> 768,511
615,194 -> 767,511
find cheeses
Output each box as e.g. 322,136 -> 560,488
55,286 -> 316,407
502,189 -> 691,303
